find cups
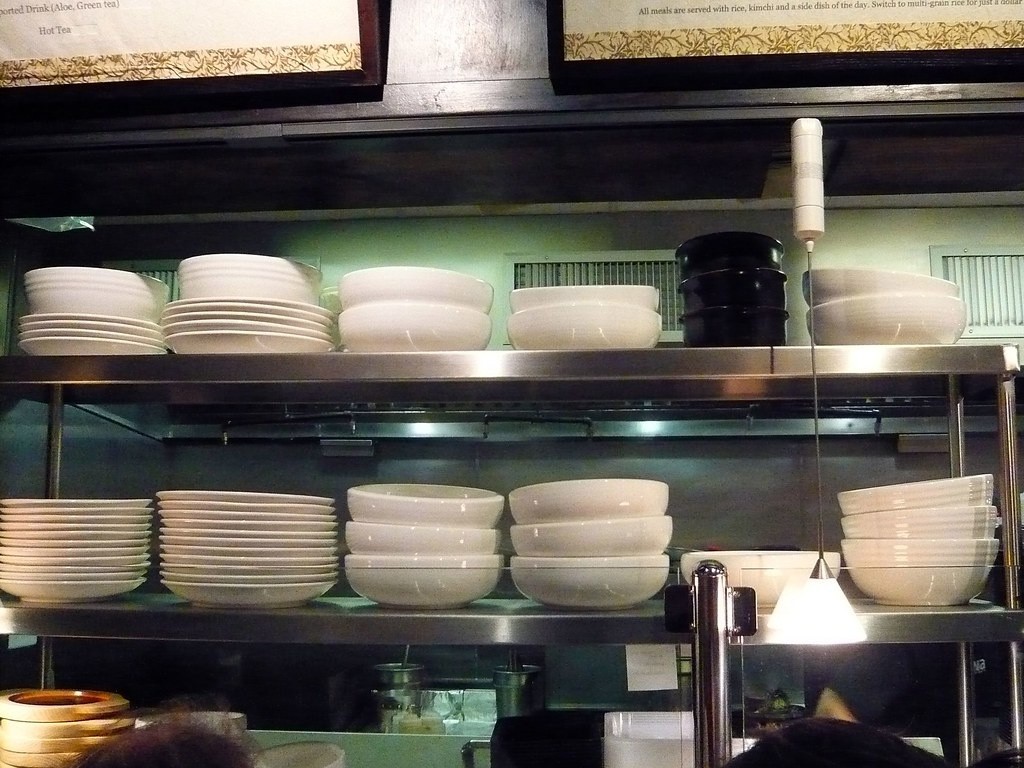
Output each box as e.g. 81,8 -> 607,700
372,662 -> 425,690
491,663 -> 541,719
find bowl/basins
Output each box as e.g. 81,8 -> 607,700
337,265 -> 495,351
249,740 -> 346,768
836,474 -> 1002,608
801,267 -> 967,345
23,266 -> 170,324
505,284 -> 663,349
674,231 -> 789,347
175,253 -> 323,308
133,709 -> 248,735
507,477 -> 674,611
679,550 -> 842,609
344,483 -> 505,610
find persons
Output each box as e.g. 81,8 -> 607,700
723,642 -> 1024,768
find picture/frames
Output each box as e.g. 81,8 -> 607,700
545,0 -> 1024,96
0,0 -> 384,102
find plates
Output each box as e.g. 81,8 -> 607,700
160,296 -> 336,355
0,687 -> 135,768
155,490 -> 339,610
17,313 -> 168,357
0,497 -> 155,603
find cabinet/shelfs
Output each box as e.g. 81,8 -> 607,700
0,344 -> 1024,768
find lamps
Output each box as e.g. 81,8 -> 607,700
765,117 -> 869,646
